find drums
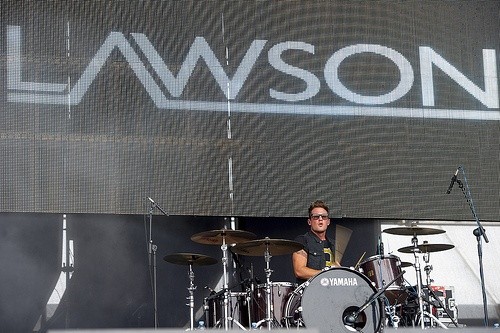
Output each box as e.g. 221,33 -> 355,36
202,291 -> 252,331
248,281 -> 299,330
356,255 -> 410,306
386,296 -> 438,331
283,266 -> 386,333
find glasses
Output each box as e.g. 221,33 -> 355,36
310,213 -> 330,220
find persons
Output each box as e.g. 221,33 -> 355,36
292,200 -> 335,287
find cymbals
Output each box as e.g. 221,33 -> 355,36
229,239 -> 305,256
396,243 -> 455,254
382,227 -> 447,236
190,229 -> 260,245
162,253 -> 219,267
400,261 -> 413,268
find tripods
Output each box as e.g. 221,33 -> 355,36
208,238 -> 281,330
410,234 -> 451,330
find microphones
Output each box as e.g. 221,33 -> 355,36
377,236 -> 380,254
148,197 -> 168,216
447,168 -> 459,193
